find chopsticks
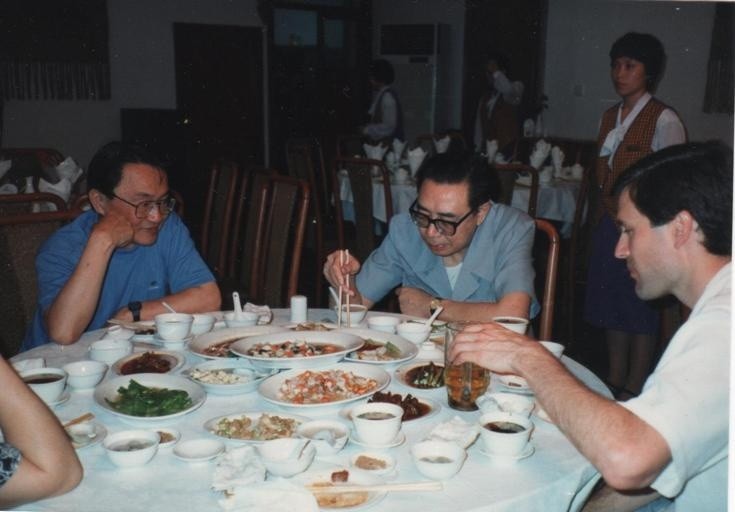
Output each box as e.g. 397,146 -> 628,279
336,249 -> 350,327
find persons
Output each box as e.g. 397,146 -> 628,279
446,140 -> 732,511
354,60 -> 406,144
16,137 -> 224,357
583,33 -> 688,404
0,349 -> 84,512
324,149 -> 538,328
474,55 -> 524,149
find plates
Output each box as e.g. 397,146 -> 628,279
111,352 -> 183,376
153,428 -> 182,448
173,438 -> 227,462
393,362 -> 446,391
261,361 -> 391,409
346,451 -> 398,478
190,324 -> 420,368
182,360 -> 278,395
495,374 -> 529,389
60,419 -> 107,450
91,373 -> 209,420
474,442 -> 537,461
287,469 -> 391,510
203,410 -> 312,443
347,433 -> 406,448
338,395 -> 440,424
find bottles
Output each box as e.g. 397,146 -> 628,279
23,176 -> 41,213
290,295 -> 308,324
536,114 -> 544,138
523,118 -> 534,138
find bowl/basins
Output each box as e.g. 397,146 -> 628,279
258,438 -> 317,478
349,404 -> 405,442
104,428 -> 158,467
411,442 -> 468,479
298,419 -> 349,456
475,413 -> 534,454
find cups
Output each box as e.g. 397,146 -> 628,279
13,357 -> 48,372
61,359 -> 110,391
89,309 -> 262,363
491,315 -> 530,337
20,367 -> 68,403
369,153 -> 586,187
538,340 -> 566,361
334,302 -> 433,348
474,392 -> 534,417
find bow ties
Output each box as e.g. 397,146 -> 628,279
600,125 -> 628,157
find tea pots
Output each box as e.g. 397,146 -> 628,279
444,320 -> 493,409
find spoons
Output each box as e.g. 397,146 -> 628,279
231,291 -> 246,321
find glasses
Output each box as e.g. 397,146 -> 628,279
408,198 -> 475,237
112,189 -> 177,220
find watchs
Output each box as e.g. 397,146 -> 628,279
428,292 -> 443,321
127,298 -> 142,322
